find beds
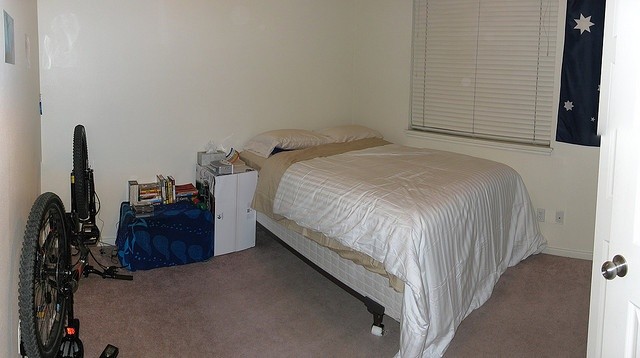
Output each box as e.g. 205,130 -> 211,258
239,126 -> 521,338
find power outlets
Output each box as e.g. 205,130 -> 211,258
536,209 -> 545,222
555,211 -> 563,224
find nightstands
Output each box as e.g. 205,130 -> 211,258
196,162 -> 258,256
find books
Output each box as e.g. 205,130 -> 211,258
128,175 -> 199,218
227,149 -> 245,173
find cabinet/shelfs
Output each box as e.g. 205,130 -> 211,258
115,202 -> 214,271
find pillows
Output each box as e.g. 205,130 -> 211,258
315,124 -> 384,143
244,128 -> 336,159
